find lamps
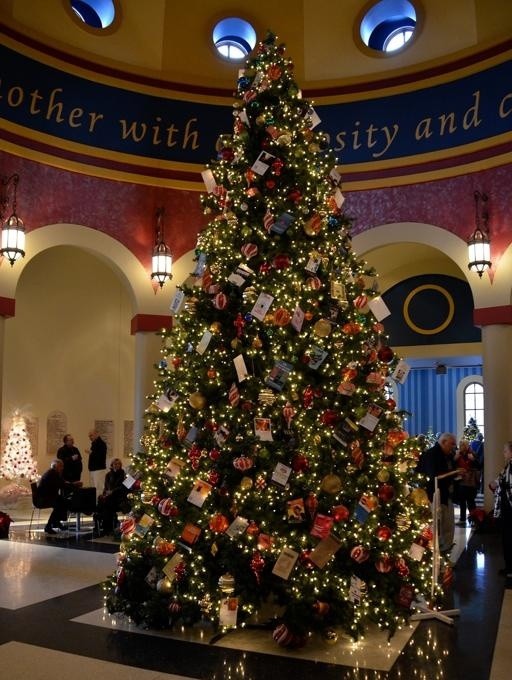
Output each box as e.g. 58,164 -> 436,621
468,190 -> 491,279
152,208 -> 173,289
1,174 -> 26,267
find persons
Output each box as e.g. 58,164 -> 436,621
37,459 -> 83,535
84,428 -> 106,498
92,458 -> 122,489
97,482 -> 134,536
55,432 -> 83,499
489,440 -> 512,578
413,430 -> 484,527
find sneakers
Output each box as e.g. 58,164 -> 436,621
44,520 -> 65,534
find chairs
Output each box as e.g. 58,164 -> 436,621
28,483 -> 115,536
